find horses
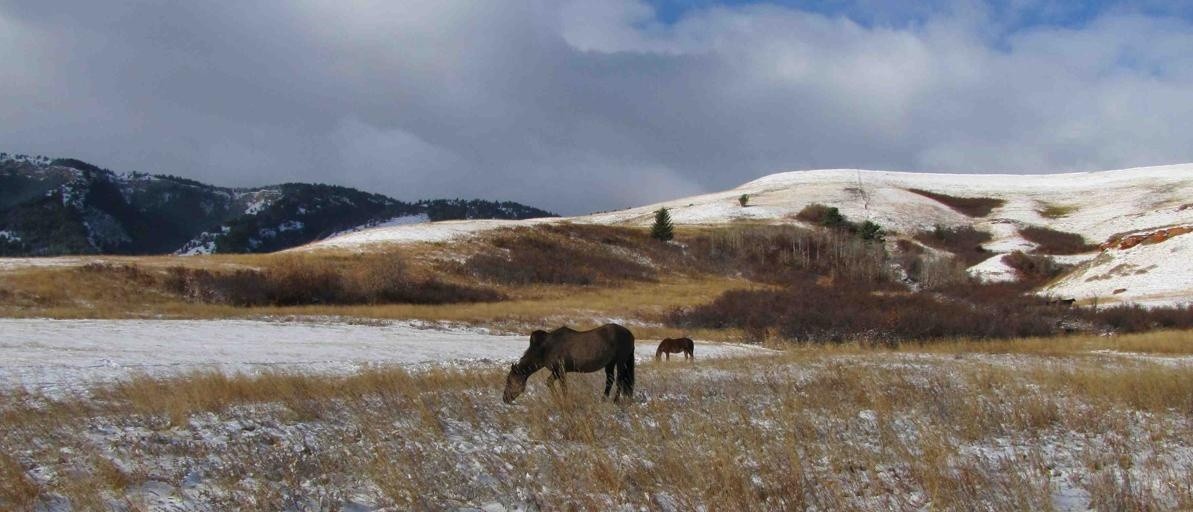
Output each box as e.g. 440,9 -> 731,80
655,335 -> 694,364
503,323 -> 635,406
